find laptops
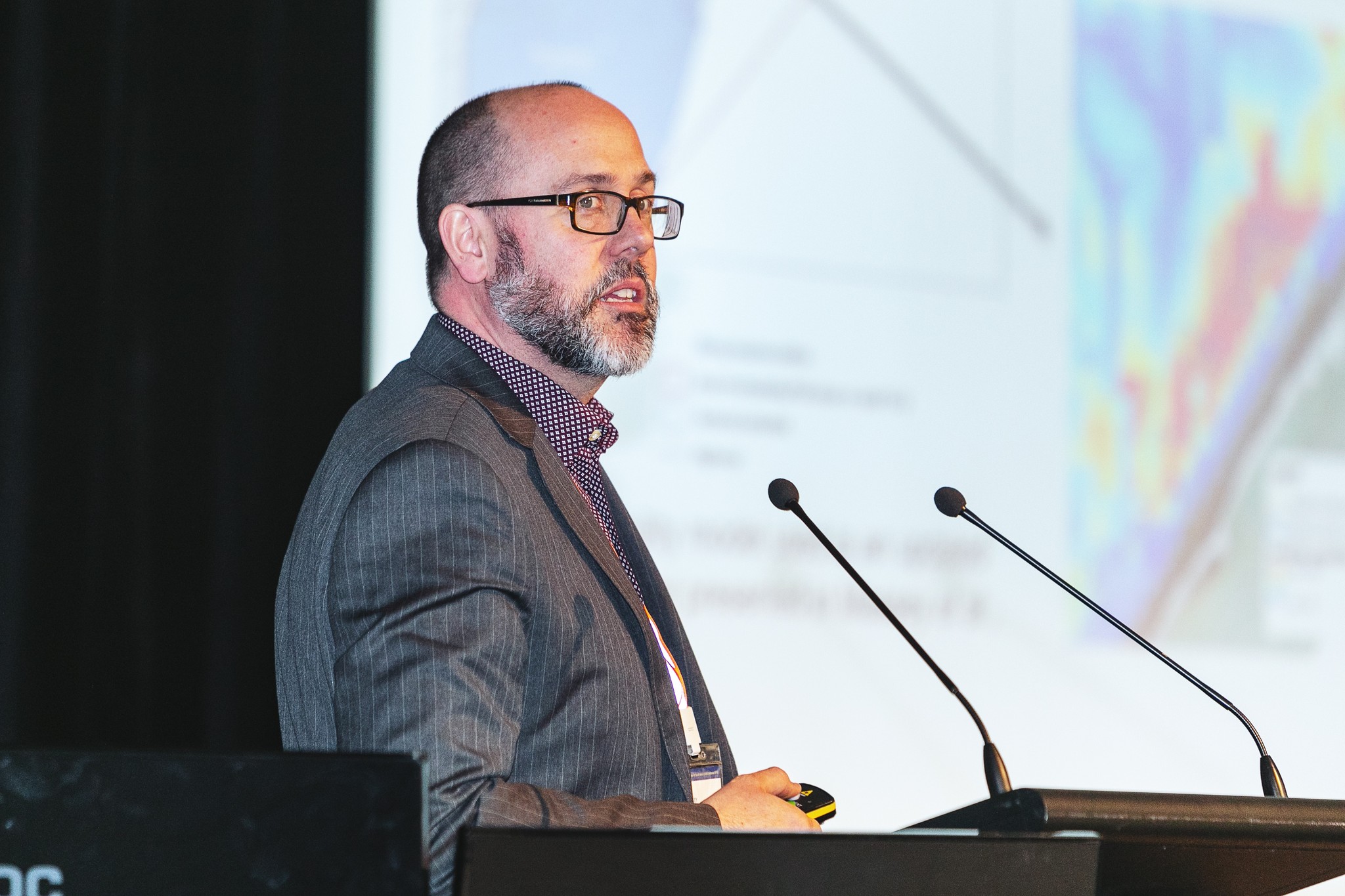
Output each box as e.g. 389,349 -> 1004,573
0,749 -> 434,895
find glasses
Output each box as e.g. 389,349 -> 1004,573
464,190 -> 683,241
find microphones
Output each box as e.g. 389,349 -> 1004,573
934,486 -> 1290,798
768,479 -> 1011,798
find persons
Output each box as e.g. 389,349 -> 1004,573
275,83 -> 823,896
